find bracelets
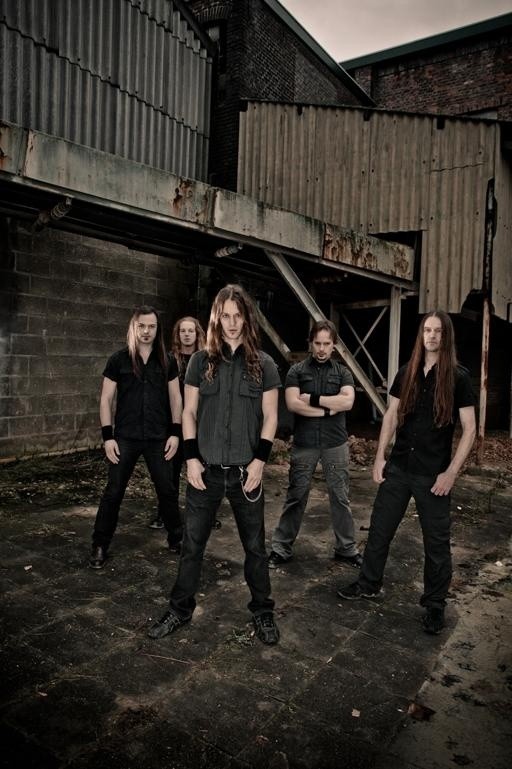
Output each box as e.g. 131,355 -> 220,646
100,426 -> 115,443
323,406 -> 331,418
253,439 -> 273,463
183,438 -> 198,462
168,422 -> 182,438
309,393 -> 322,408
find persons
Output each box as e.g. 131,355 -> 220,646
266,320 -> 365,570
148,313 -> 221,530
335,307 -> 480,638
147,282 -> 284,645
88,304 -> 186,569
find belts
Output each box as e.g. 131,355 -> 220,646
205,464 -> 247,469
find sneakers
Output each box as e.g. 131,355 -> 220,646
422,606 -> 446,635
252,611 -> 280,644
147,507 -> 223,529
336,580 -> 380,601
147,610 -> 193,640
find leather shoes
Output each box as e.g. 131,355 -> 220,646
267,550 -> 291,569
168,537 -> 183,555
334,550 -> 364,568
89,543 -> 108,569
